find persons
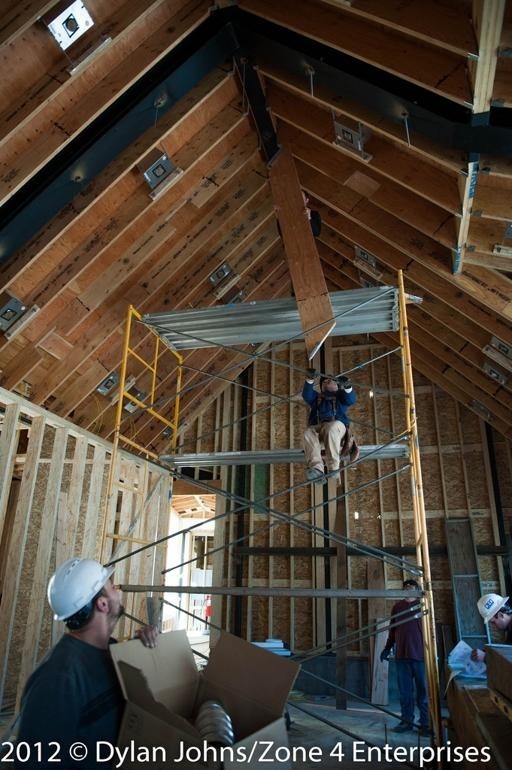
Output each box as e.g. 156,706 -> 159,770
301,367 -> 358,485
470,593 -> 511,662
1,557 -> 158,769
380,578 -> 430,737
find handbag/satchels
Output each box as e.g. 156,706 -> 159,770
339,427 -> 359,462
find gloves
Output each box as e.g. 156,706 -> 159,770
338,376 -> 351,389
306,368 -> 316,379
381,639 -> 395,663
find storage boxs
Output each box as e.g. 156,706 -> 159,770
108,623 -> 298,770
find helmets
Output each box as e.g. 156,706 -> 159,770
47,558 -> 117,621
477,593 -> 510,624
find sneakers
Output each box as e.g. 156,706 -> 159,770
391,721 -> 412,733
306,469 -> 327,486
